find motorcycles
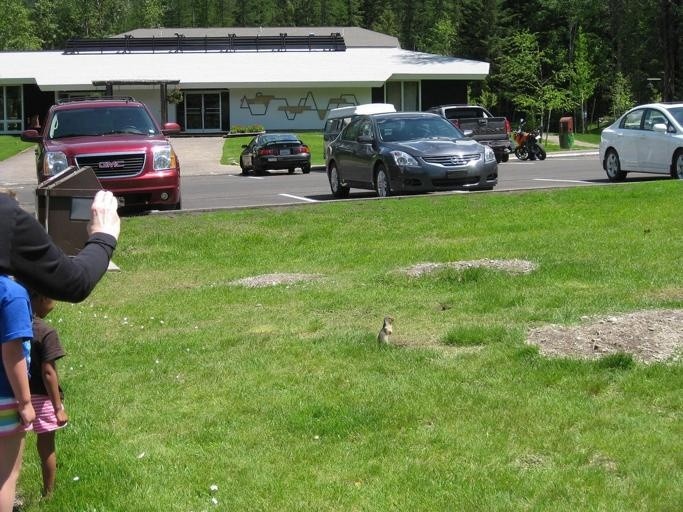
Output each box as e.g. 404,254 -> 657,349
512,119 -> 546,162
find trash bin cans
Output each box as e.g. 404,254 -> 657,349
559,116 -> 574,149
35,164 -> 104,257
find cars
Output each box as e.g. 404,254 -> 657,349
20,96 -> 182,212
325,113 -> 495,194
238,133 -> 313,174
599,103 -> 682,180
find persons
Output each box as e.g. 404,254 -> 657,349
0,272 -> 37,512
16,280 -> 69,496
0,190 -> 122,303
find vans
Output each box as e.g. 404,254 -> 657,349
325,104 -> 395,157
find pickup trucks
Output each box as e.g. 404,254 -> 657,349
428,103 -> 511,162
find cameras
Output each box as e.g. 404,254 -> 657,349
70,195 -> 94,223
